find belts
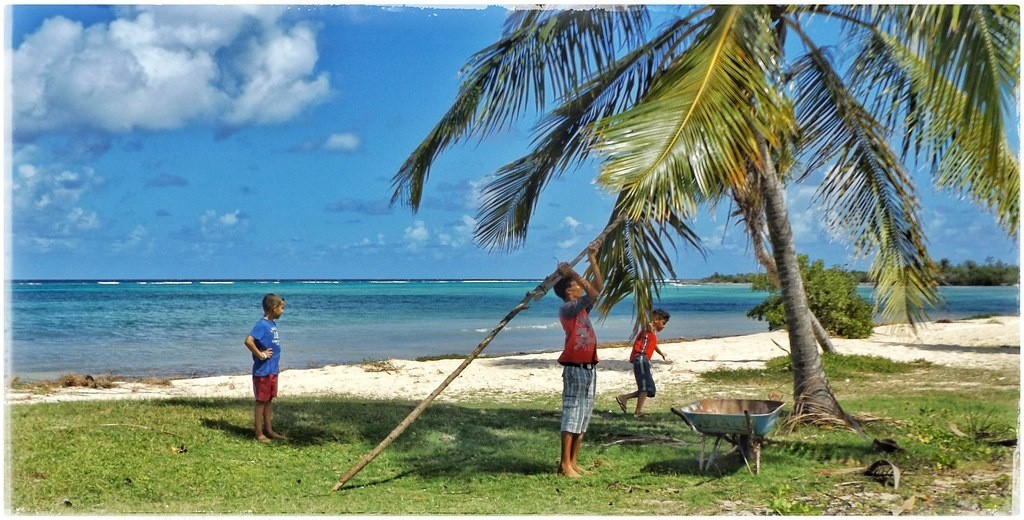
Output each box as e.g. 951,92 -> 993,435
565,362 -> 595,370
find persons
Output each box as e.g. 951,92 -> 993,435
244,292 -> 288,444
615,307 -> 670,419
552,237 -> 603,482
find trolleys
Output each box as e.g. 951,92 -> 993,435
670,397 -> 789,476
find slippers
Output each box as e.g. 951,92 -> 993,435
616,396 -> 627,414
635,414 -> 648,419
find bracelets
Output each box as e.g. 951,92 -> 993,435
259,351 -> 267,361
